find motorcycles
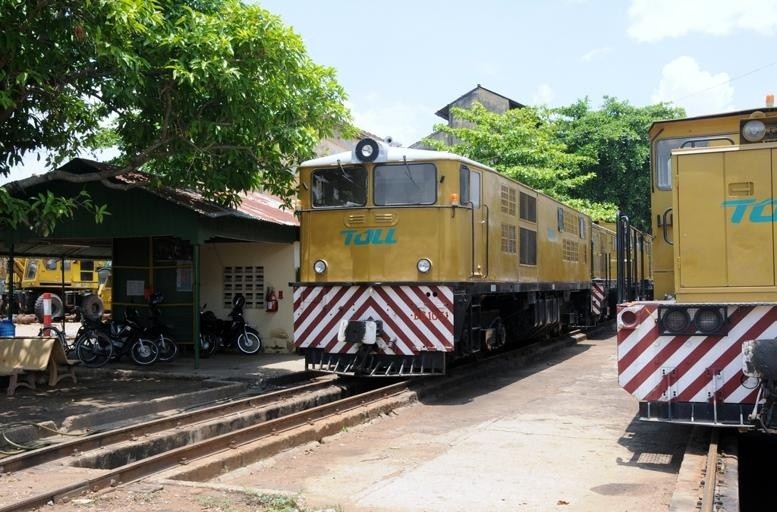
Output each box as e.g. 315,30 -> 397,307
38,297 -> 177,365
199,295 -> 261,357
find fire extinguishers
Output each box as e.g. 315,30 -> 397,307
266,287 -> 277,312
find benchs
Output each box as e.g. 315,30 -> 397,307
0,336 -> 82,396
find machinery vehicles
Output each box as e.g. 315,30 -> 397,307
1,256 -> 111,322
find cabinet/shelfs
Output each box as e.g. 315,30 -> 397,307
111,236 -> 200,370
